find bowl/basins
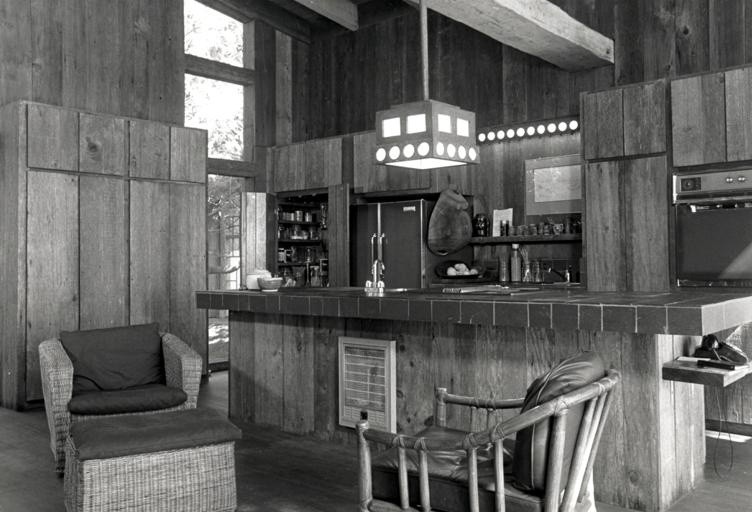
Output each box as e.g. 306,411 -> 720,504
257,277 -> 283,293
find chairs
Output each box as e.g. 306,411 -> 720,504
37,319 -> 205,479
354,347 -> 623,512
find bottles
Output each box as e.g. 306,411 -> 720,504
510,245 -> 523,284
278,247 -> 285,264
500,219 -> 509,237
564,216 -> 573,234
532,260 -> 544,284
281,210 -> 312,222
521,260 -> 534,284
278,227 -> 289,239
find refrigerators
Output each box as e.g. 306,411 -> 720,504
349,199 -> 473,288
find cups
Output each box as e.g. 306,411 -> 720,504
507,223 -> 563,235
309,226 -> 316,240
499,255 -> 510,283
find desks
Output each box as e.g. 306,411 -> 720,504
194,285 -> 751,511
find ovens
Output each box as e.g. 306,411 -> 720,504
671,165 -> 752,291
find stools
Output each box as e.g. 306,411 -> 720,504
61,405 -> 246,511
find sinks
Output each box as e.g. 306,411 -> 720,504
384,287 -> 418,292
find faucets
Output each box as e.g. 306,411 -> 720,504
373,259 -> 387,288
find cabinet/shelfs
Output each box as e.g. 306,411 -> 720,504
353,129 -> 475,199
265,136 -> 351,198
2,99 -> 211,413
671,63 -> 752,169
277,199 -> 328,267
579,76 -> 673,160
582,156 -> 672,293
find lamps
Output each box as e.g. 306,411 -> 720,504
371,1 -> 479,173
